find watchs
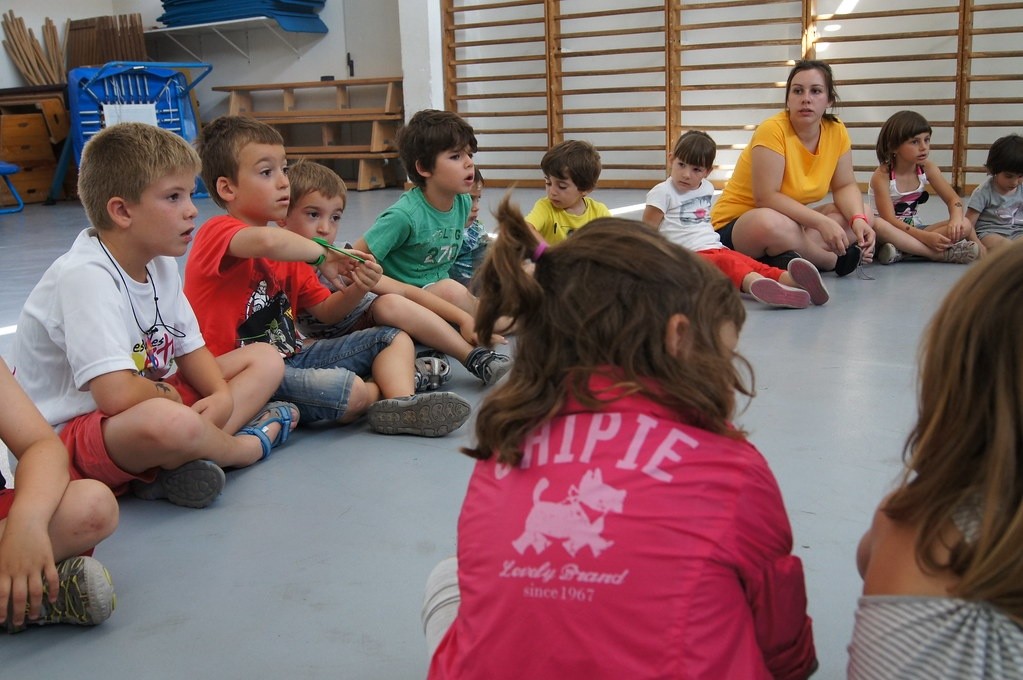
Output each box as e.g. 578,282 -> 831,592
304,238 -> 366,266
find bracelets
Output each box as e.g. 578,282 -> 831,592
849,214 -> 869,229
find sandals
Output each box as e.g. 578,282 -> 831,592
130,460 -> 226,509
413,356 -> 451,392
365,392 -> 472,439
235,401 -> 301,461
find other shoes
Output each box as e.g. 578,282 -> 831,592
833,243 -> 863,276
748,277 -> 810,309
787,258 -> 830,305
463,346 -> 513,386
877,242 -> 905,265
767,249 -> 802,271
944,241 -> 979,264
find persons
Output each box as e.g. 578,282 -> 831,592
964,134 -> 1023,261
521,140 -> 612,246
642,130 -> 830,310
5,123 -> 300,508
422,217 -> 819,679
865,111 -> 979,264
353,108 -> 521,337
0,357 -> 120,633
711,61 -> 876,277
183,115 -> 472,439
270,162 -> 514,387
844,236 -> 1023,678
448,167 -> 534,297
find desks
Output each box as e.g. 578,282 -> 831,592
68,60 -> 213,198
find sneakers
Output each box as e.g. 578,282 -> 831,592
0,555 -> 116,626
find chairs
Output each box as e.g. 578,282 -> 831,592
0,162 -> 24,213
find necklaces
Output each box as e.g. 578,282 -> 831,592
95,232 -> 186,371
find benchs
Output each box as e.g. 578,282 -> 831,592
200,77 -> 403,191
